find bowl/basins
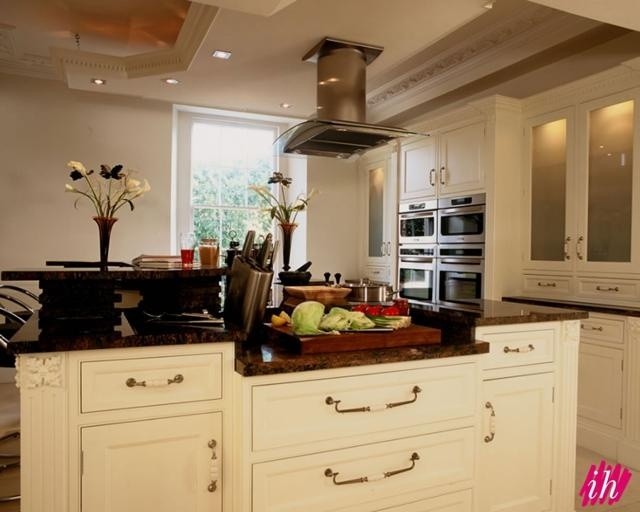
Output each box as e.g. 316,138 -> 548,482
278,271 -> 312,284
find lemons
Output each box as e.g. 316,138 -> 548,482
271,314 -> 286,326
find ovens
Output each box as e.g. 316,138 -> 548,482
396,192 -> 487,308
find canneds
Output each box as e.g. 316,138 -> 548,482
199,238 -> 219,269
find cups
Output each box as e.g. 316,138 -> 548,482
179,231 -> 195,268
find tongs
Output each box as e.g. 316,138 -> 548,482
269,36 -> 430,160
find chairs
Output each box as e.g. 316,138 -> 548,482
0,285 -> 41,502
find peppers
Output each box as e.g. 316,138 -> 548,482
353,298 -> 409,316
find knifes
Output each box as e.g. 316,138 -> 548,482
241,229 -> 276,268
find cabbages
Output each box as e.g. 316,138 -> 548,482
291,300 -> 326,337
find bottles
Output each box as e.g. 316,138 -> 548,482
198,238 -> 221,267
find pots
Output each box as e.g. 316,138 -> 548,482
344,277 -> 401,302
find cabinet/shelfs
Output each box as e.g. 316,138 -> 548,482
474,320 -> 561,510
359,159 -> 394,283
516,86 -> 639,470
235,355 -> 486,511
397,121 -> 486,201
66,342 -> 236,511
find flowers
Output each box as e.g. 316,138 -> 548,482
245,171 -> 322,225
65,161 -> 152,218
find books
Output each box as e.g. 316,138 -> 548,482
136,261 -> 198,269
132,252 -> 198,264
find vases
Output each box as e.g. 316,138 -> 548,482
93,217 -> 119,267
277,224 -> 299,271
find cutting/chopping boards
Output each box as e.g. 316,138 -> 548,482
262,322 -> 443,356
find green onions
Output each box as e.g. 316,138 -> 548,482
366,315 -> 412,331
